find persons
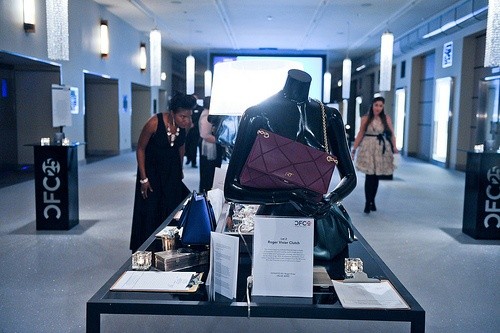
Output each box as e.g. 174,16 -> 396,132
352,97 -> 398,214
129,94 -> 197,255
183,94 -> 227,194
224,69 -> 357,281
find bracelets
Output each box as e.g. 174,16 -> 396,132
352,149 -> 356,155
140,178 -> 148,184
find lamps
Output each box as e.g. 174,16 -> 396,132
46,0 -> 69,61
149,19 -> 161,88
101,20 -> 109,57
324,43 -> 332,103
185,24 -> 195,94
342,36 -> 351,98
25,0 -> 36,29
204,44 -> 213,96
140,42 -> 147,69
379,21 -> 394,91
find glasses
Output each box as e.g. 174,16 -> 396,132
178,114 -> 190,119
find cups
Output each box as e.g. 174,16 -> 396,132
161,235 -> 180,251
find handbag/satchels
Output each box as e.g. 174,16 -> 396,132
210,115 -> 242,147
177,188 -> 218,245
239,128 -> 338,200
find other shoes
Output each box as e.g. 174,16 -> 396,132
364,199 -> 376,213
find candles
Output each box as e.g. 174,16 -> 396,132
131,250 -> 152,269
344,257 -> 364,276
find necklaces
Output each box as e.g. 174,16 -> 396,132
167,121 -> 180,147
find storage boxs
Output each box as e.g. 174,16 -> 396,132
155,249 -> 211,271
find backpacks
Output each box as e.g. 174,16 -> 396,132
257,187 -> 358,281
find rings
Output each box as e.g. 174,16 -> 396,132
141,191 -> 144,194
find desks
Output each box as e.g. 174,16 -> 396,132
85,189 -> 426,333
